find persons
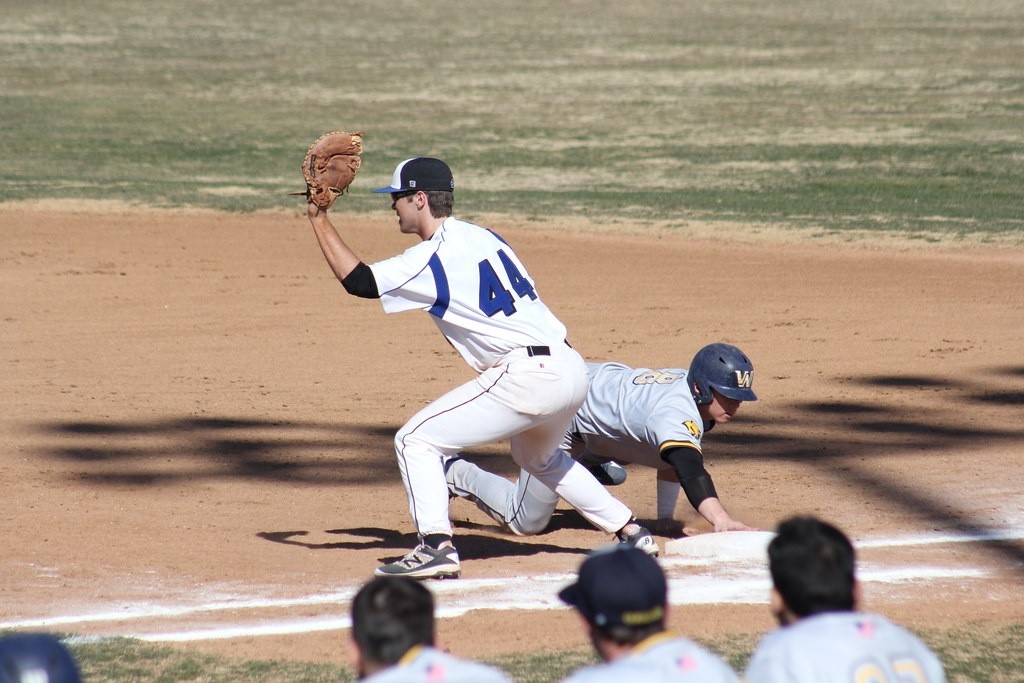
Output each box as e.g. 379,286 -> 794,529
442,343 -> 761,537
348,573 -> 512,683
0,633 -> 83,683
744,514 -> 948,683
558,550 -> 743,682
301,131 -> 658,579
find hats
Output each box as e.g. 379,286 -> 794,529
372,157 -> 455,192
557,549 -> 668,641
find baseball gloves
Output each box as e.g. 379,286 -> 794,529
288,129 -> 367,218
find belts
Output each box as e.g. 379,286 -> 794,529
528,339 -> 571,355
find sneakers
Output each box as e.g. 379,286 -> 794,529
374,537 -> 461,580
571,453 -> 627,485
441,453 -> 462,499
613,526 -> 659,559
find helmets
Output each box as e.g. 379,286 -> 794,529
687,343 -> 758,404
0,635 -> 79,683
